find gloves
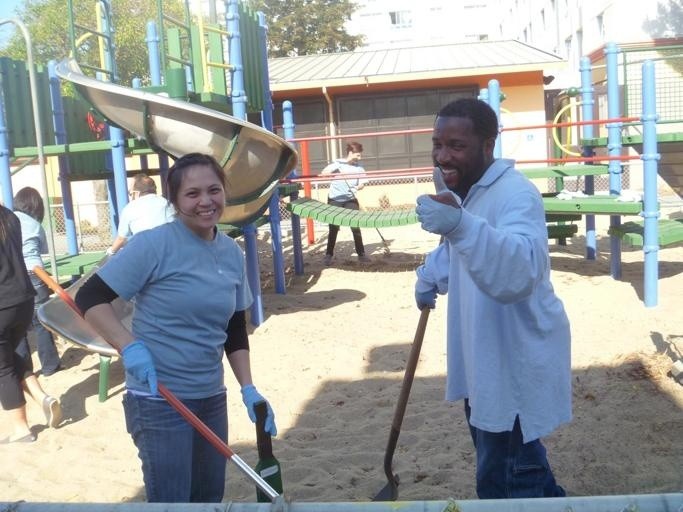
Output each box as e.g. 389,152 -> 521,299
415,288 -> 437,310
240,384 -> 277,436
120,342 -> 157,394
416,167 -> 462,235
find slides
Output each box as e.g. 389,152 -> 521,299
33,62 -> 299,403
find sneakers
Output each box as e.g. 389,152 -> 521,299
0,433 -> 36,444
359,256 -> 371,262
43,396 -> 60,427
325,255 -> 332,264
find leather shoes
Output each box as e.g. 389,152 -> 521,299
43,363 -> 66,376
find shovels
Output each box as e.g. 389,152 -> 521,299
372,306 -> 429,502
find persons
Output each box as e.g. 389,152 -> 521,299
11,186 -> 60,377
0,202 -> 63,445
414,97 -> 573,497
75,153 -> 277,503
103,174 -> 179,258
319,141 -> 372,265
